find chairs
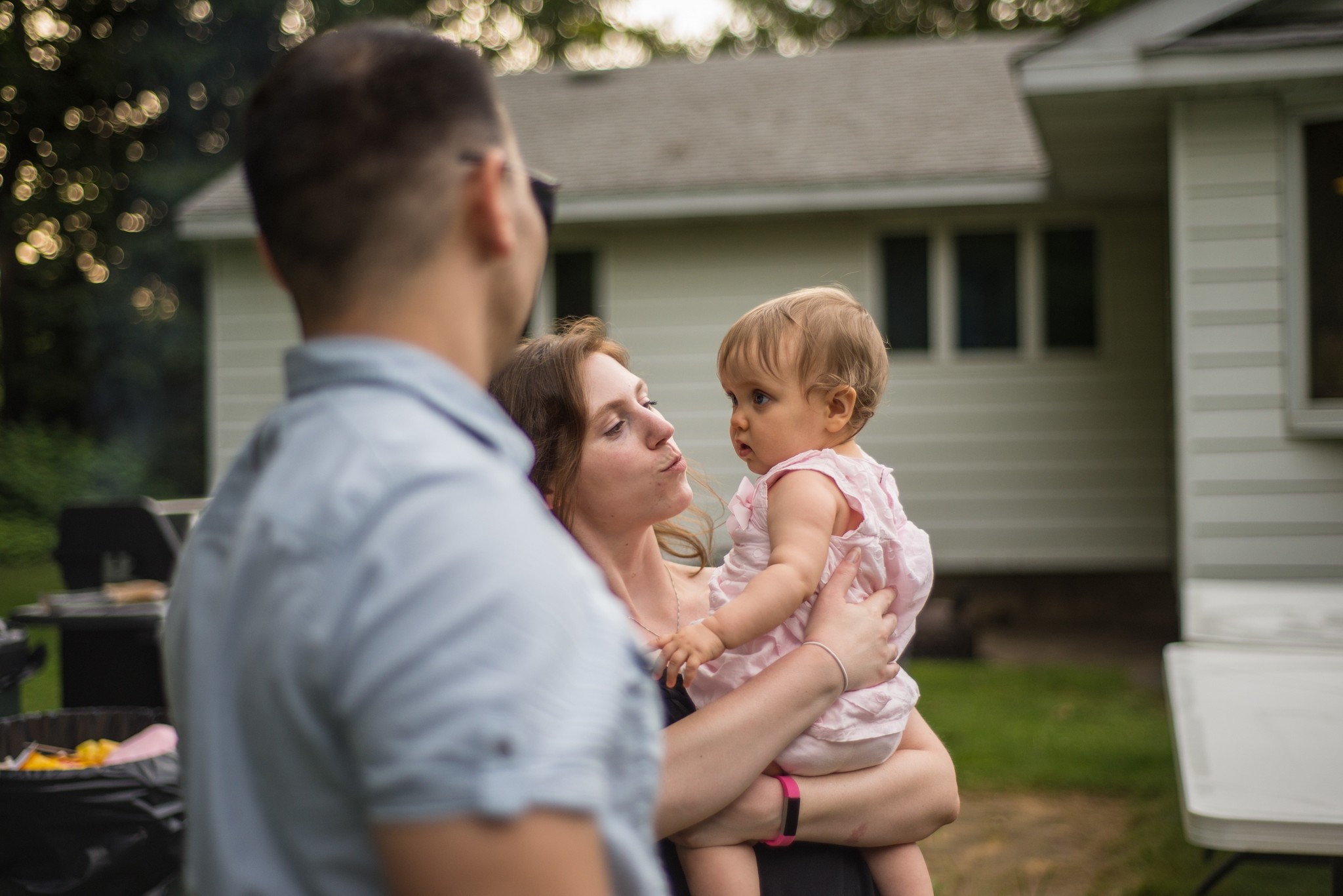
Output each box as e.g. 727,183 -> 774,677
58,499 -> 182,596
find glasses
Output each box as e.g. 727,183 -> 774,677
455,145 -> 558,231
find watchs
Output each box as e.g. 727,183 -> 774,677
760,774 -> 802,850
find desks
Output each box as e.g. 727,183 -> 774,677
7,590 -> 170,711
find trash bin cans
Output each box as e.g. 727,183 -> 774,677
0,709 -> 186,896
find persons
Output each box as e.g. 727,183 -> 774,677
159,20 -> 678,896
495,288 -> 960,896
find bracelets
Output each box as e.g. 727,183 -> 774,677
800,641 -> 849,696
